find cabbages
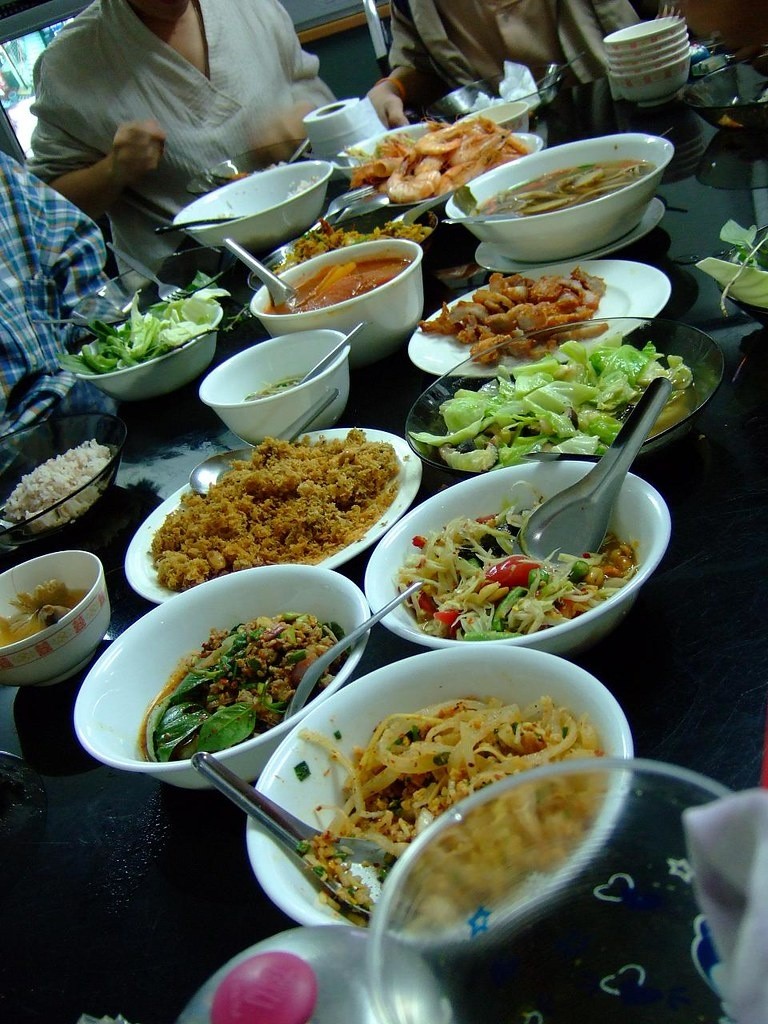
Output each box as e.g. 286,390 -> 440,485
406,332 -> 693,473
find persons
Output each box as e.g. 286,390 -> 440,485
365,0 -> 768,185
0,151 -> 127,484
25,0 -> 337,297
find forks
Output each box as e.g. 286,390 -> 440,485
107,242 -> 224,304
303,185 -> 378,235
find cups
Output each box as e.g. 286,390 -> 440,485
367,758 -> 735,1024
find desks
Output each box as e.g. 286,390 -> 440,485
0,66 -> 768,1024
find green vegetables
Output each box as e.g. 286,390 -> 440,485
56,269 -> 231,377
146,611 -> 350,761
694,217 -> 768,317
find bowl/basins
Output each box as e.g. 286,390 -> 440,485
404,317 -> 724,476
0,412 -> 125,685
73,564 -> 370,787
197,328 -> 350,448
445,133 -> 674,263
717,224 -> 768,327
334,55 -> 566,181
71,246 -> 237,402
602,15 -> 768,131
250,209 -> 438,365
173,137 -> 334,249
364,460 -> 671,654
246,645 -> 634,958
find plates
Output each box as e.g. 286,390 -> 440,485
409,197 -> 672,376
386,134 -> 544,206
125,428 -> 421,605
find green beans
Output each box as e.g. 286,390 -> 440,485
463,560 -> 590,642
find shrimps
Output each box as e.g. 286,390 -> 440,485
342,107 -> 532,204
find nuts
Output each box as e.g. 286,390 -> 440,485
558,543 -> 635,617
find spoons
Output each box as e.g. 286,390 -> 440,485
513,377 -> 672,561
334,193 -> 390,223
284,582 -> 422,721
188,387 -> 338,495
222,237 -> 298,307
191,752 -> 397,917
391,191 -> 457,223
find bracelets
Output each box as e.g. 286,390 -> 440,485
375,77 -> 407,107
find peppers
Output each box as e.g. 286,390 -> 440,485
412,535 -> 538,625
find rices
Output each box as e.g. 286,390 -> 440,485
0,437 -> 114,534
282,178 -> 313,201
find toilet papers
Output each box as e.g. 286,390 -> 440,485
301,94 -> 391,183
